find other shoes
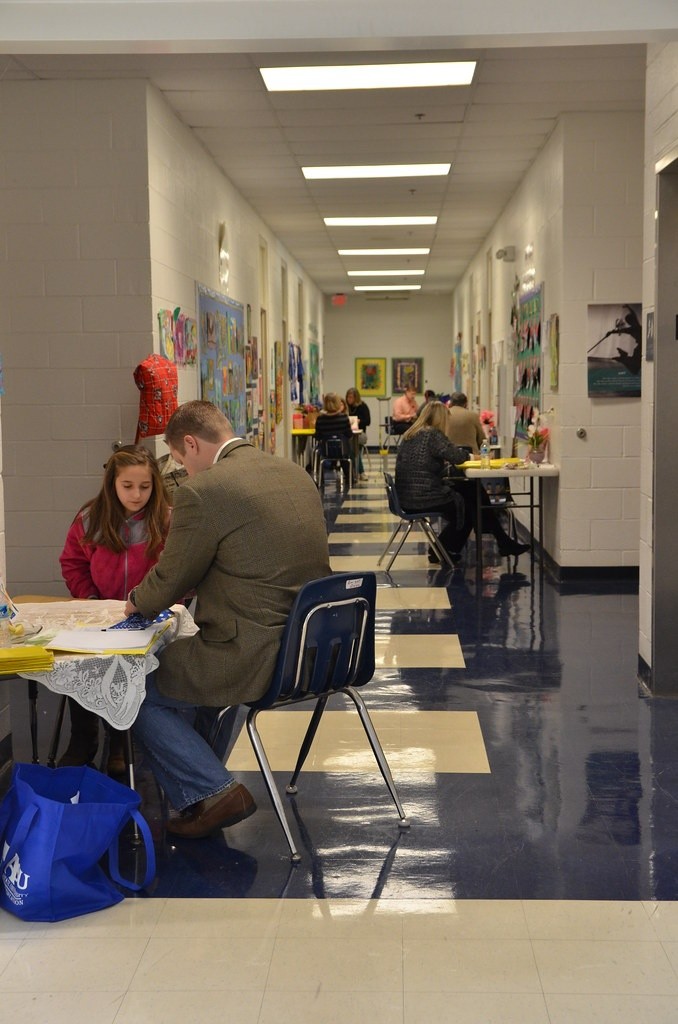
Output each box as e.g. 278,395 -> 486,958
107,733 -> 145,775
56,740 -> 99,769
499,539 -> 531,557
360,472 -> 369,482
346,478 -> 357,484
429,551 -> 460,562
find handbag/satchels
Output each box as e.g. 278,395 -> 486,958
0,761 -> 159,922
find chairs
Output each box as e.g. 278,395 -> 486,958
207,572 -> 410,864
316,432 -> 353,488
377,472 -> 455,573
383,416 -> 403,450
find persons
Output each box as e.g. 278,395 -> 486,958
316,392 -> 358,492
392,387 -> 420,435
416,390 -> 436,426
346,388 -> 371,480
444,391 -> 486,454
124,400 -> 332,835
57,444 -> 185,788
395,401 -> 530,563
439,395 -> 451,408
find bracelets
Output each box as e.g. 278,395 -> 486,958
130,590 -> 136,607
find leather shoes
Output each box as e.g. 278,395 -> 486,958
164,782 -> 257,838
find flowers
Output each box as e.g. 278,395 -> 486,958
514,423 -> 550,451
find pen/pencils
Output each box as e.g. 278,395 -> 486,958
101,628 -> 145,631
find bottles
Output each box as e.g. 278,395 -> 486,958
0,593 -> 12,650
480,439 -> 490,469
293,413 -> 303,429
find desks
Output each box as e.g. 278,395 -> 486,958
291,429 -> 362,479
0,597 -> 187,847
463,458 -> 559,577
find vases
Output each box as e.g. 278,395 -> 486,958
529,446 -> 545,463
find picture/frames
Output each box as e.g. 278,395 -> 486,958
391,357 -> 425,396
354,357 -> 386,397
